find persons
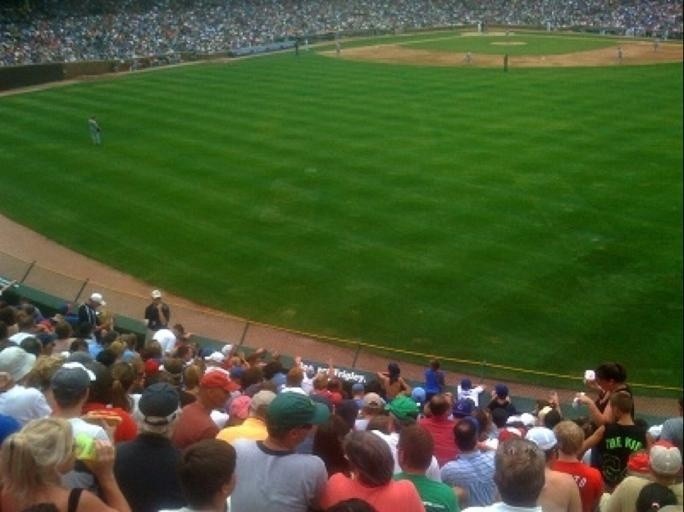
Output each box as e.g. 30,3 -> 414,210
88,114 -> 103,145
0,288 -> 684,512
0,0 -> 683,78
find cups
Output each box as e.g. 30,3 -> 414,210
73,432 -> 101,461
583,370 -> 595,384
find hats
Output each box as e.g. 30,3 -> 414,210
494,384 -> 509,395
0,346 -> 36,383
90,293 -> 106,306
138,382 -> 179,417
50,361 -> 96,391
151,290 -> 161,300
647,421 -> 682,474
362,385 -> 427,422
201,370 -> 242,392
265,391 -> 330,429
480,413 -> 558,450
453,379 -> 472,416
